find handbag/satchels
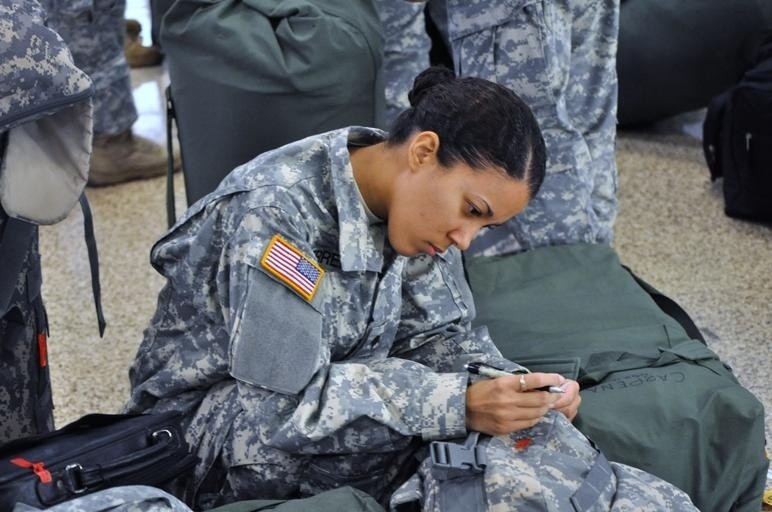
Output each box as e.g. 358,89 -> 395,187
0,412 -> 198,510
701,59 -> 772,232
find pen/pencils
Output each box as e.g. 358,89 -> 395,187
464,362 -> 566,393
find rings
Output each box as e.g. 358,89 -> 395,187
519,374 -> 527,394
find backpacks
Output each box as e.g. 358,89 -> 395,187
205,486 -> 383,511
164,0 -> 391,235
391,241 -> 768,512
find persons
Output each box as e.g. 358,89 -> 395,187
366,0 -> 622,257
122,64 -> 706,512
26,0 -> 183,190
